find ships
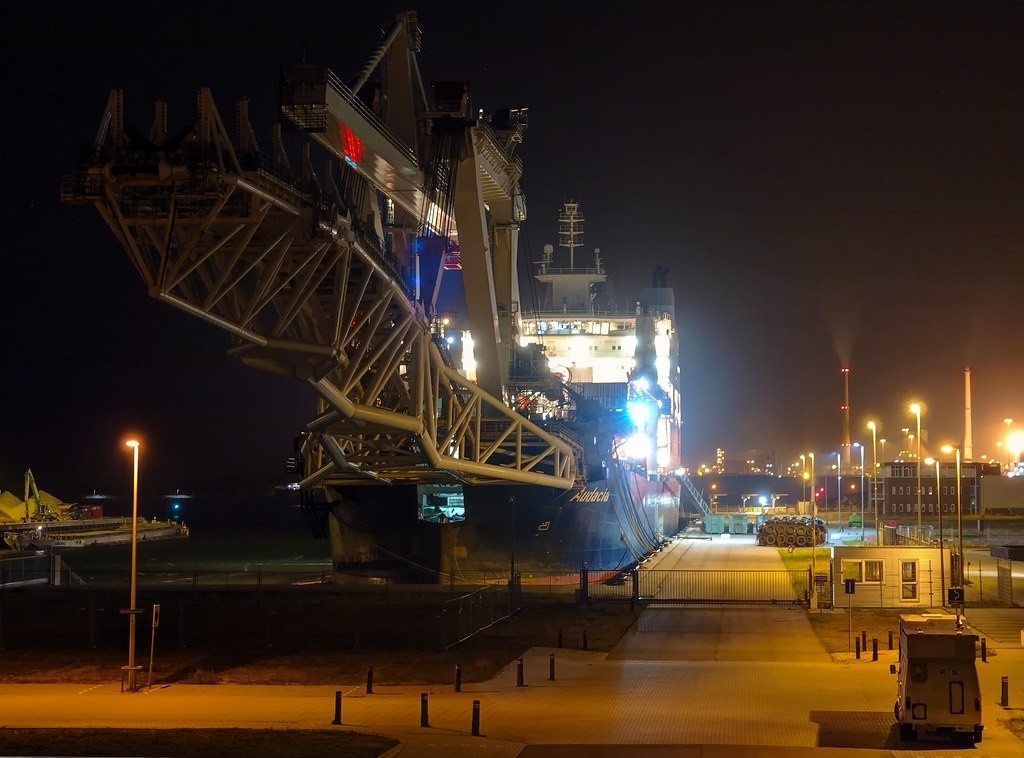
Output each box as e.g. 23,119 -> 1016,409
48,1 -> 681,581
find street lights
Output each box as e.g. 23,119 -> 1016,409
879,415 -> 1021,503
832,450 -> 842,535
924,456 -> 943,547
941,444 -> 966,565
865,418 -> 880,527
124,432 -> 141,692
798,450 -> 817,521
852,440 -> 865,539
906,397 -> 927,541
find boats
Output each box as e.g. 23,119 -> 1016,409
1,467 -> 189,572
